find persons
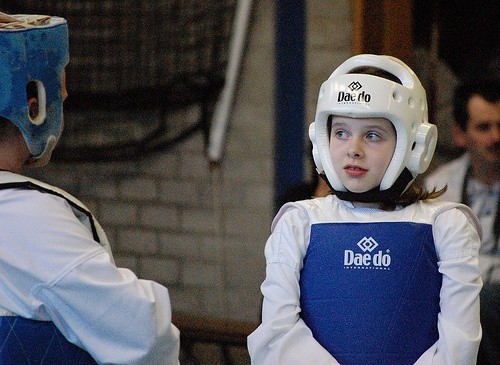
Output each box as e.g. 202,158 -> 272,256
422,64 -> 500,365
245,52 -> 484,365
0,12 -> 181,365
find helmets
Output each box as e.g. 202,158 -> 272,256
308,54 -> 438,199
0,11 -> 69,169
